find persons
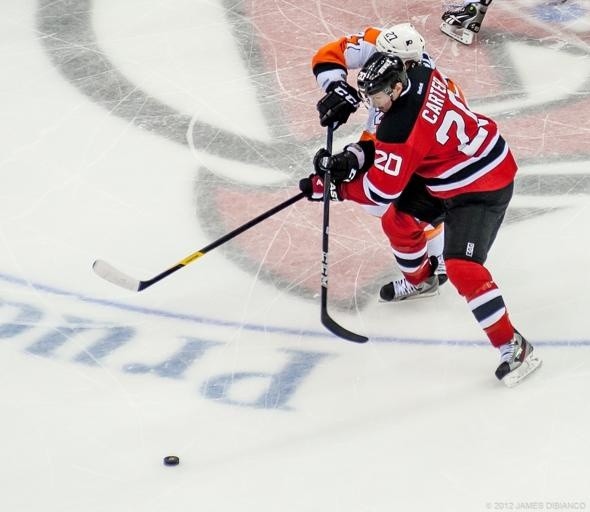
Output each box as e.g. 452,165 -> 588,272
441,0 -> 493,33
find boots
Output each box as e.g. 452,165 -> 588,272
379,254 -> 448,301
441,3 -> 487,32
495,329 -> 533,380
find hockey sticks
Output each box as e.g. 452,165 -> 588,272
93,192 -> 306,291
321,122 -> 368,343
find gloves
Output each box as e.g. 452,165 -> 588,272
317,81 -> 362,129
297,144 -> 363,202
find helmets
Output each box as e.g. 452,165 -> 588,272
356,22 -> 425,95
